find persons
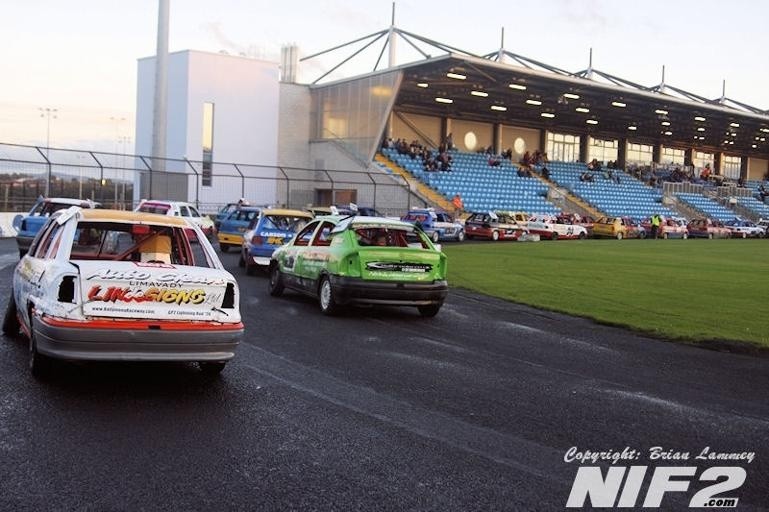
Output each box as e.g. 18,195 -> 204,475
451,192 -> 463,217
650,213 -> 663,239
382,131 -> 769,204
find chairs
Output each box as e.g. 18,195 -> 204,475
376,148 -> 769,226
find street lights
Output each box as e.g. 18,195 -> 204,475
76,115 -> 134,209
37,105 -> 60,198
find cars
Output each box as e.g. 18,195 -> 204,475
214,198 -> 468,323
